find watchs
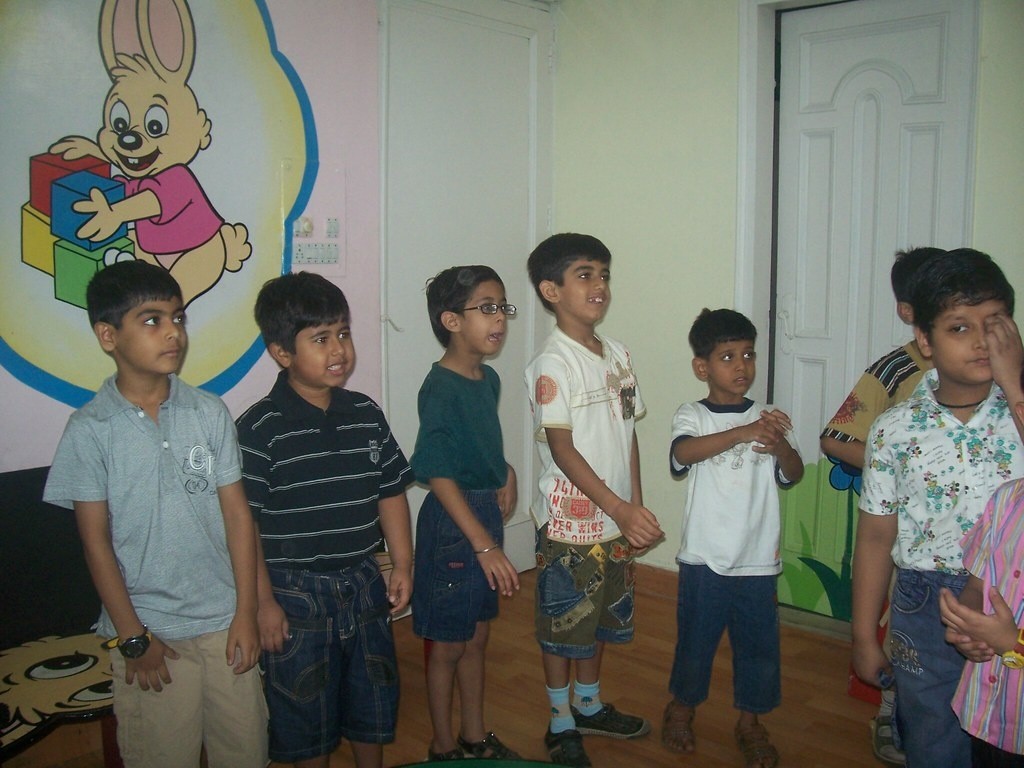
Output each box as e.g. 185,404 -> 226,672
100,625 -> 152,659
1002,630 -> 1024,671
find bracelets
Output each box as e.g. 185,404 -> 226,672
473,544 -> 501,555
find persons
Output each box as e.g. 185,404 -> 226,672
527,233 -> 651,768
820,246 -> 949,471
850,249 -> 1024,768
938,476 -> 1024,768
41,258 -> 270,767
657,307 -> 804,768
410,264 -> 521,762
234,271 -> 414,768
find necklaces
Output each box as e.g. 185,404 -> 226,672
938,398 -> 987,408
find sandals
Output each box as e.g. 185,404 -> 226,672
457,730 -> 522,761
424,740 -> 471,762
735,722 -> 777,768
868,714 -> 907,764
661,700 -> 697,755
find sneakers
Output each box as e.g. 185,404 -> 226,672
546,720 -> 591,768
570,701 -> 651,739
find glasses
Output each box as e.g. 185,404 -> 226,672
453,302 -> 516,316
